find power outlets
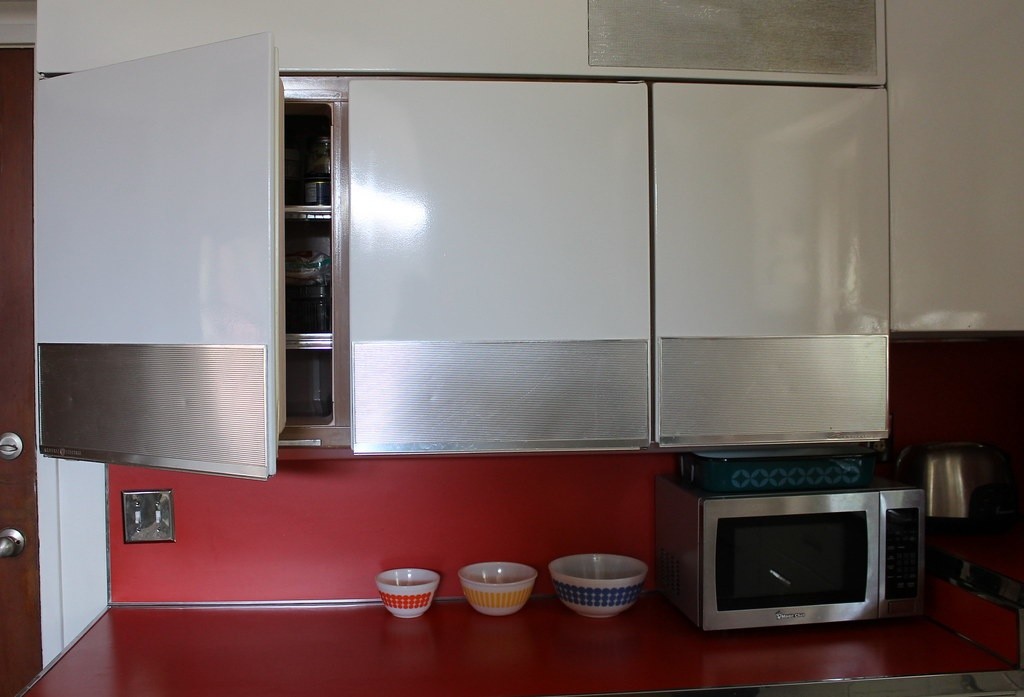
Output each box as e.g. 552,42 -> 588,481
120,488 -> 176,544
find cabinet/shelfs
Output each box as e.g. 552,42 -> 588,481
651,78 -> 891,451
34,31 -> 655,482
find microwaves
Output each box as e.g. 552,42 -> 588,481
654,473 -> 927,633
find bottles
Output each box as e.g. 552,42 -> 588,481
303,137 -> 331,205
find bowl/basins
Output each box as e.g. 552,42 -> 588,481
548,553 -> 648,619
375,568 -> 440,619
457,561 -> 538,616
